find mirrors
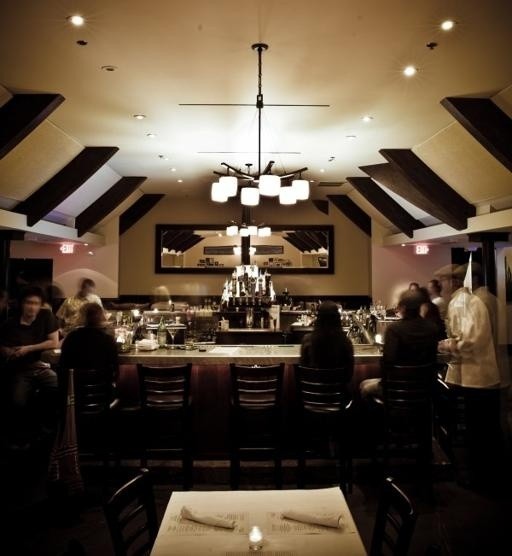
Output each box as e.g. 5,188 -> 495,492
155,223 -> 336,274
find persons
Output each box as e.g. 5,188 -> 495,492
1,277 -> 121,418
300,302 -> 354,409
360,264 -> 512,460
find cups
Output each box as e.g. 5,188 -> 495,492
218,320 -> 229,329
248,532 -> 265,551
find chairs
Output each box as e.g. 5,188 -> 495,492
63,363 -> 124,492
227,363 -> 284,486
135,362 -> 196,491
101,466 -> 158,552
294,362 -> 356,496
368,478 -> 418,555
371,359 -> 431,485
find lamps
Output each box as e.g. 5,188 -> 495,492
225,162 -> 273,240
209,41 -> 313,207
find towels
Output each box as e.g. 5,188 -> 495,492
181,506 -> 234,529
281,507 -> 344,530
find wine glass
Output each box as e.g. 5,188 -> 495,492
169,329 -> 179,350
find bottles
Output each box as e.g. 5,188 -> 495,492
117,312 -> 132,353
194,297 -> 212,317
260,317 -> 264,328
246,306 -> 254,328
212,302 -> 218,311
339,304 -> 386,327
157,314 -> 168,348
207,327 -> 217,343
